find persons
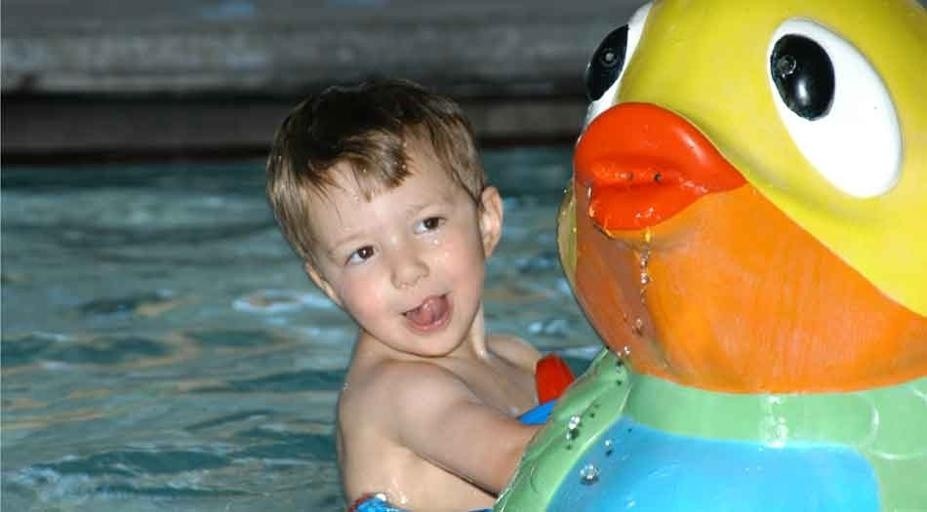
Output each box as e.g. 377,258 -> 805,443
266,76 -> 546,512
